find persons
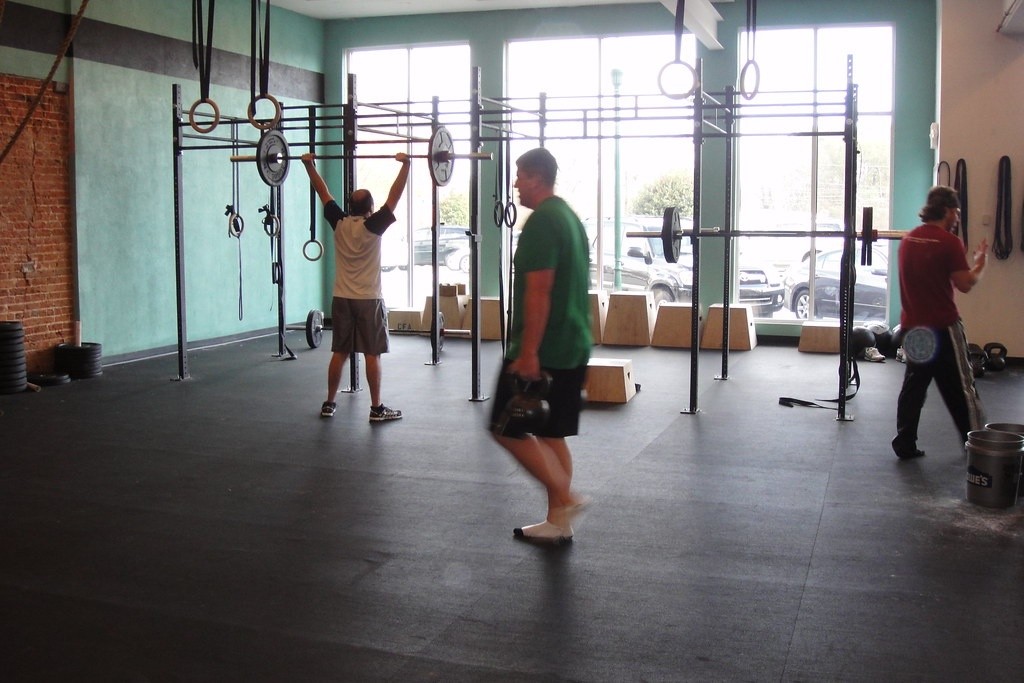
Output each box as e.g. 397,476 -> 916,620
301,153 -> 410,423
891,186 -> 986,459
486,148 -> 591,542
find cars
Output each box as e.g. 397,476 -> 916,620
379,225 -> 472,273
782,245 -> 889,323
447,230 -> 522,276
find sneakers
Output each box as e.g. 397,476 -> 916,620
864,346 -> 885,362
895,346 -> 906,364
321,400 -> 338,417
369,403 -> 403,421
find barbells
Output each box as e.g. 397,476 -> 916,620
229,125 -> 494,187
285,309 -> 472,352
625,206 -> 910,267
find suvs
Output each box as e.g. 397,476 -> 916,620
577,214 -> 785,319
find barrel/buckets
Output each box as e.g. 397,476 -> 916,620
984,422 -> 1024,498
965,430 -> 1024,508
